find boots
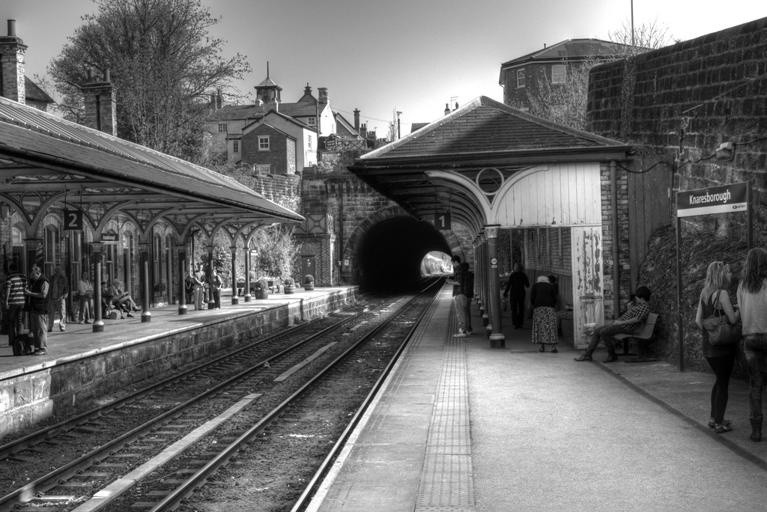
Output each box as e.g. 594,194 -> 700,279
749,415 -> 763,442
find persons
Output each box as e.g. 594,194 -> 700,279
530,276 -> 559,353
461,263 -> 474,335
78,271 -> 93,324
574,286 -> 652,363
47,267 -> 69,332
24,266 -> 49,355
696,262 -> 741,432
100,280 -> 142,319
5,262 -> 29,355
446,255 -> 468,337
737,247 -> 767,441
184,263 -> 222,311
503,263 -> 530,329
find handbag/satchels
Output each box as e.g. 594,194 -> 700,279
702,312 -> 741,345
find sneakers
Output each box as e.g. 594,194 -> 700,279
453,328 -> 473,338
573,353 -> 593,361
33,347 -> 49,356
538,345 -> 546,353
708,416 -> 730,428
78,304 -> 141,324
714,422 -> 732,434
550,346 -> 558,353
603,354 -> 618,363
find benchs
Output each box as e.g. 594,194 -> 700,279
613,312 -> 665,362
